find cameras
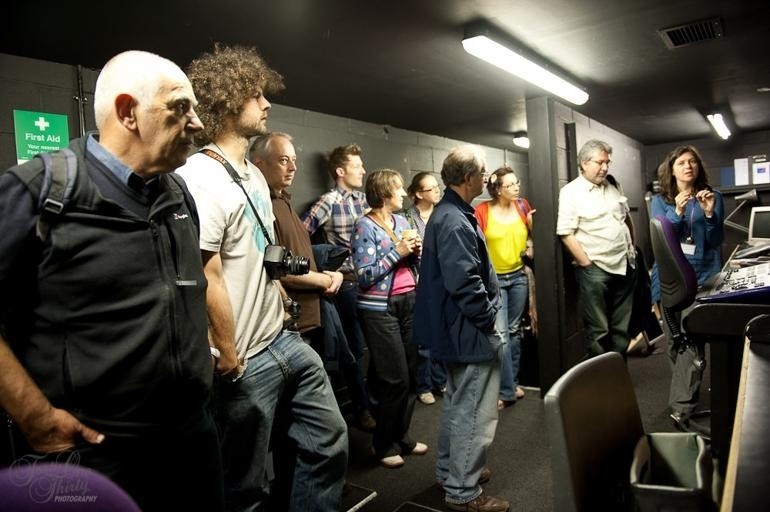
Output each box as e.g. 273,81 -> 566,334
262,243 -> 311,277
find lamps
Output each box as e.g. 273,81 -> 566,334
723,189 -> 758,234
513,131 -> 530,149
461,25 -> 590,106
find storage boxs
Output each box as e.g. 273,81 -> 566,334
629,432 -> 707,512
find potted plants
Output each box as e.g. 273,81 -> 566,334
401,217 -> 418,243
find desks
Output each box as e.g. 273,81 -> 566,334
679,244 -> 770,459
719,314 -> 770,512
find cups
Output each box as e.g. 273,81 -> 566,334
401,229 -> 417,239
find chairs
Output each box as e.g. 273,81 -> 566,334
0,463 -> 140,512
543,350 -> 719,512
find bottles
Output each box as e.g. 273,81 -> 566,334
652,181 -> 660,193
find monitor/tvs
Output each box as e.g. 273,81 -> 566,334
748,206 -> 770,246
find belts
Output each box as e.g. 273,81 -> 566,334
78,411 -> 214,441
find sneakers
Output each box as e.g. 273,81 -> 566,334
410,441 -> 430,456
372,447 -> 405,469
444,489 -> 512,512
418,388 -> 438,407
478,466 -> 491,486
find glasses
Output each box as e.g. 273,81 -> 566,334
498,178 -> 522,189
589,159 -> 614,166
419,184 -> 442,194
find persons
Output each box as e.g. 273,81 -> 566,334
1,44 -> 724,511
